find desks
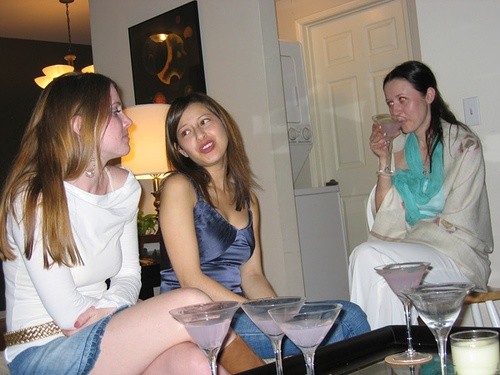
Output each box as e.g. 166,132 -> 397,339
234,324 -> 500,375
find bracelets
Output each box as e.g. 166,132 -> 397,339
376,170 -> 395,176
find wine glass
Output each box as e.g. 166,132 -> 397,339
400,282 -> 477,375
267,302 -> 344,375
370,114 -> 407,176
372,261 -> 431,363
168,300 -> 242,375
238,296 -> 308,375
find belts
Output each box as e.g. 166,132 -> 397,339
4,319 -> 64,347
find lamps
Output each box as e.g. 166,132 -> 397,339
34,0 -> 95,90
119,104 -> 179,252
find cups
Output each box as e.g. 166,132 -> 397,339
449,330 -> 500,375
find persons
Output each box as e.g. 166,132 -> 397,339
348,60 -> 494,331
158,92 -> 370,361
0,72 -> 265,375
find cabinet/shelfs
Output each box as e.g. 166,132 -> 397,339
280,40 -> 310,126
295,192 -> 353,302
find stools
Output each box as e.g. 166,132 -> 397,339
465,286 -> 500,328
